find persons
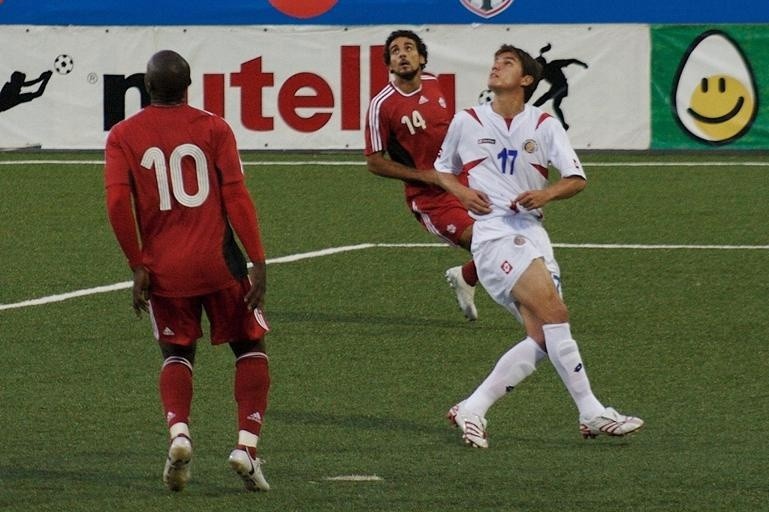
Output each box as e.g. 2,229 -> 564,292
533,43 -> 589,130
106,50 -> 274,493
364,30 -> 480,322
0,70 -> 52,112
433,43 -> 645,450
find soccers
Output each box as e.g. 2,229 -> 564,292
54,55 -> 73,74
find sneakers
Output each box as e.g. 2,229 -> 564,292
447,401 -> 489,450
228,449 -> 271,493
578,405 -> 644,440
445,263 -> 481,323
162,437 -> 193,492
